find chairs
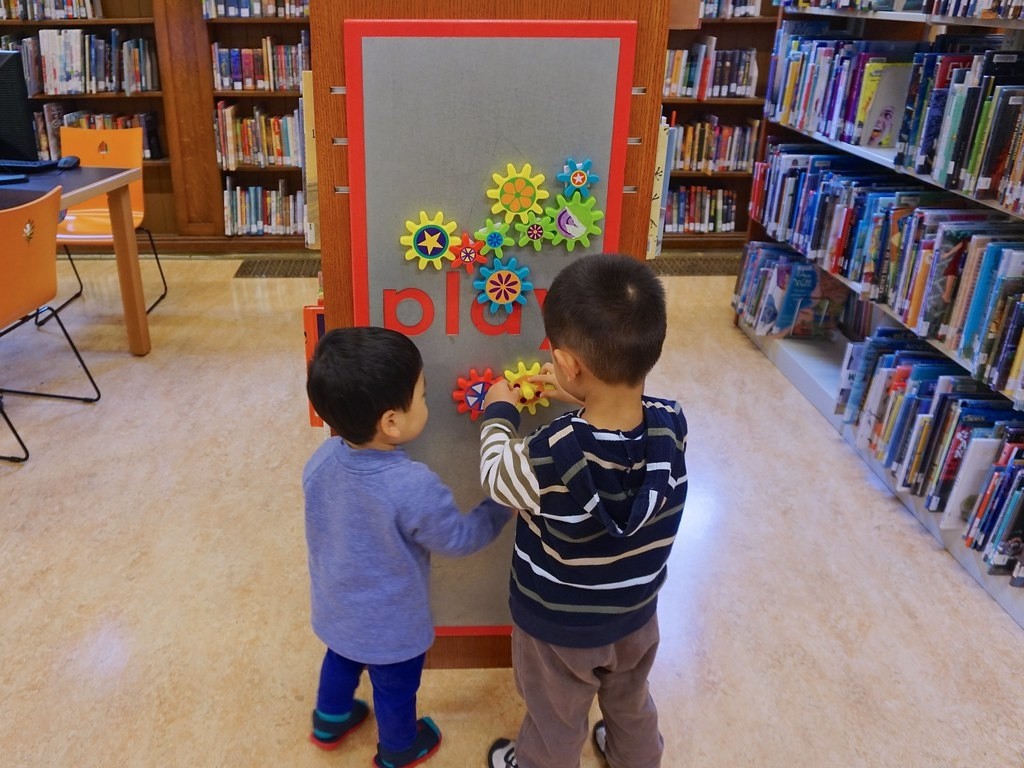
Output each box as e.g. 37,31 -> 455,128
0,182 -> 102,465
33,126 -> 169,327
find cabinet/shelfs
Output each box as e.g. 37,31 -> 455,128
729,0 -> 1024,632
0,0 -> 315,252
654,0 -> 785,258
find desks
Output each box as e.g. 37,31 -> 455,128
0,161 -> 152,360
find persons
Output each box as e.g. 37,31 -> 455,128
478,253 -> 690,768
301,326 -> 517,768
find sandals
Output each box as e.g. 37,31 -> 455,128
371,720 -> 443,767
309,701 -> 370,750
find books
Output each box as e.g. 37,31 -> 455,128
646,0 -> 763,260
0,0 -> 162,163
730,0 -> 1024,590
199,0 -> 322,248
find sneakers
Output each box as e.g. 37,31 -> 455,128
486,737 -> 520,768
594,720 -> 609,755
